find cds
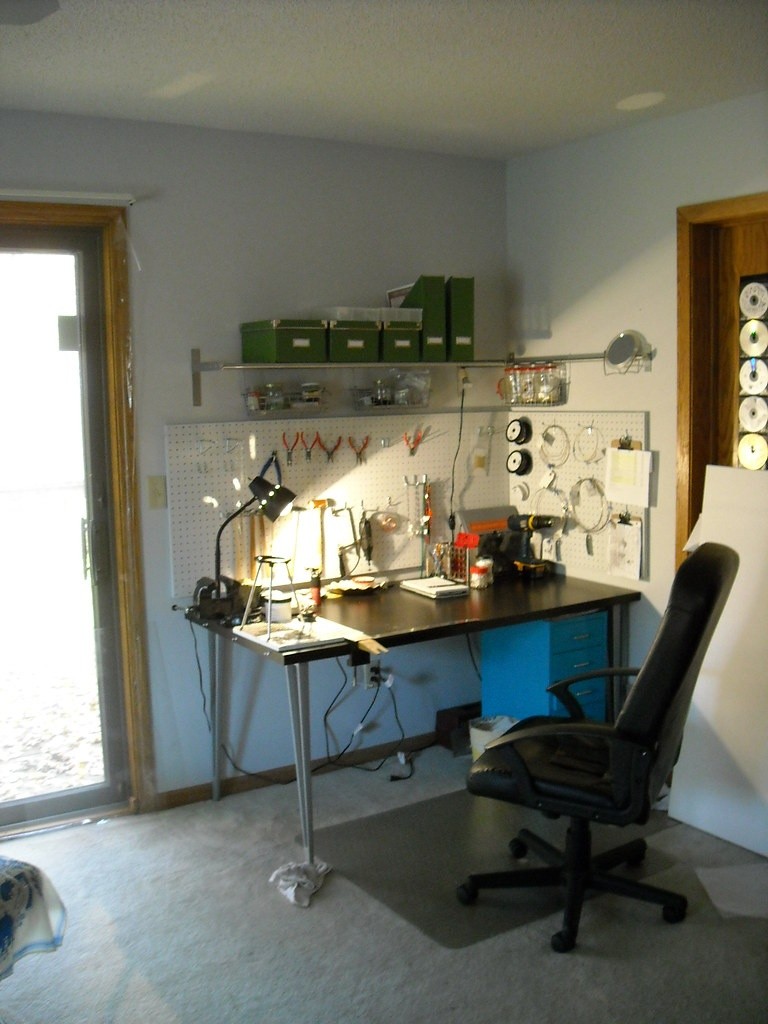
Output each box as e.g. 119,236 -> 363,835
739,281 -> 768,470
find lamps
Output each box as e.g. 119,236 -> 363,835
192,475 -> 297,620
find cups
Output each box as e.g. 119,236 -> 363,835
470,566 -> 487,588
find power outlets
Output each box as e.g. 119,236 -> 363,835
364,659 -> 381,689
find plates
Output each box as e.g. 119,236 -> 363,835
327,580 -> 385,595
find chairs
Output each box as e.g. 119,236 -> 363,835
465,540 -> 740,955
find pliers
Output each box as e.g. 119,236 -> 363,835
260,449 -> 283,485
404,430 -> 422,457
281,432 -> 370,467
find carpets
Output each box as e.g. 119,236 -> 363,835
295,789 -> 681,950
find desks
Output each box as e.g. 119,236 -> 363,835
184,573 -> 642,867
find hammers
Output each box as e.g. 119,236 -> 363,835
218,505 -> 307,580
308,499 -> 329,570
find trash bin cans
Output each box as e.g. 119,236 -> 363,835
467,715 -> 519,764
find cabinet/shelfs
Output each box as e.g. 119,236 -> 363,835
480,612 -> 607,721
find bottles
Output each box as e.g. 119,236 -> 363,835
507,514 -> 554,532
301,383 -> 321,405
266,384 -> 284,410
374,380 -> 388,405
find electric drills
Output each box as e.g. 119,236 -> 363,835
477,531 -> 513,581
507,514 -> 555,578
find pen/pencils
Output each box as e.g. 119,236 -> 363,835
427,543 -> 445,577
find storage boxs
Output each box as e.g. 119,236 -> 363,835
446,276 -> 476,363
327,320 -> 382,363
310,306 -> 379,322
239,319 -> 328,364
379,307 -> 423,321
400,270 -> 448,363
381,320 -> 423,363
